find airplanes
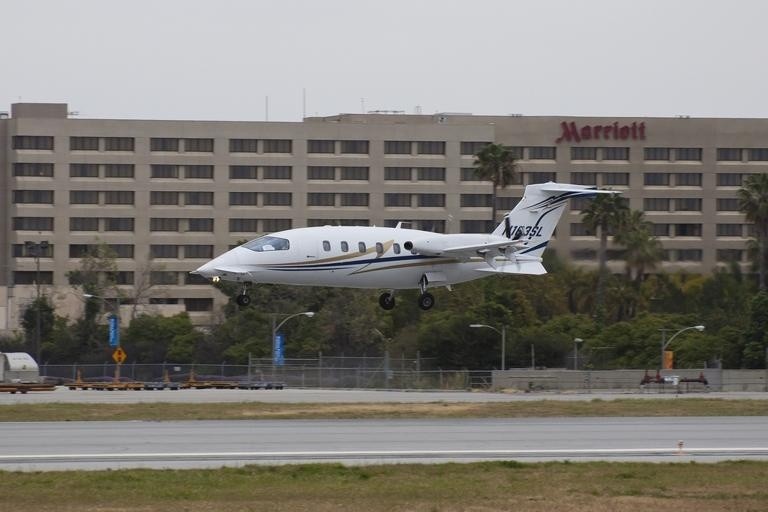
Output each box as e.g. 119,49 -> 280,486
188,183 -> 623,310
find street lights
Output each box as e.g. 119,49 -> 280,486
273,313 -> 315,367
469,324 -> 505,368
82,293 -> 121,380
661,324 -> 704,369
574,339 -> 585,368
24,239 -> 49,367
